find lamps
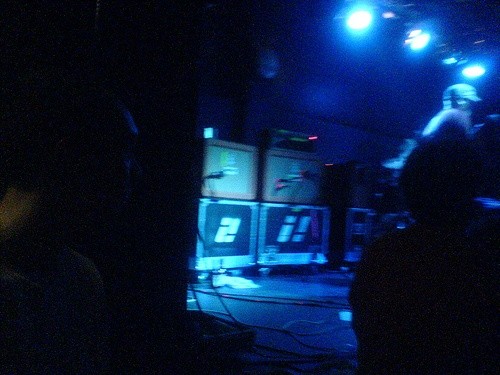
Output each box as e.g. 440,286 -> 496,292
462,60 -> 486,78
336,1 -> 374,33
403,25 -> 429,50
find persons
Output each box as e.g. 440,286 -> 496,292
346,135 -> 500,375
422,83 -> 483,137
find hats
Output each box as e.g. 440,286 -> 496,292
442,83 -> 482,102
